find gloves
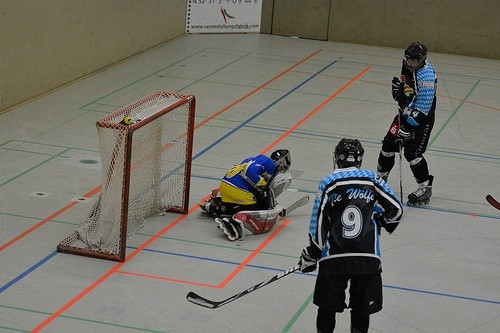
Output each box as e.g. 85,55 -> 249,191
297,246 -> 321,273
391,77 -> 402,101
398,124 -> 411,143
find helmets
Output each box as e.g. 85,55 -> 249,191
405,42 -> 427,71
333,138 -> 365,168
270,149 -> 291,173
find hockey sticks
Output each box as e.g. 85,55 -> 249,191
485,195 -> 500,211
185,264 -> 301,309
399,111 -> 403,208
278,193 -> 311,216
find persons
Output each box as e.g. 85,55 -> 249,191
296,138 -> 403,333
197,149 -> 292,241
376,42 -> 438,206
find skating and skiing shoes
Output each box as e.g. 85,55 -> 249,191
198,202 -> 210,212
215,218 -> 242,240
407,175 -> 434,206
